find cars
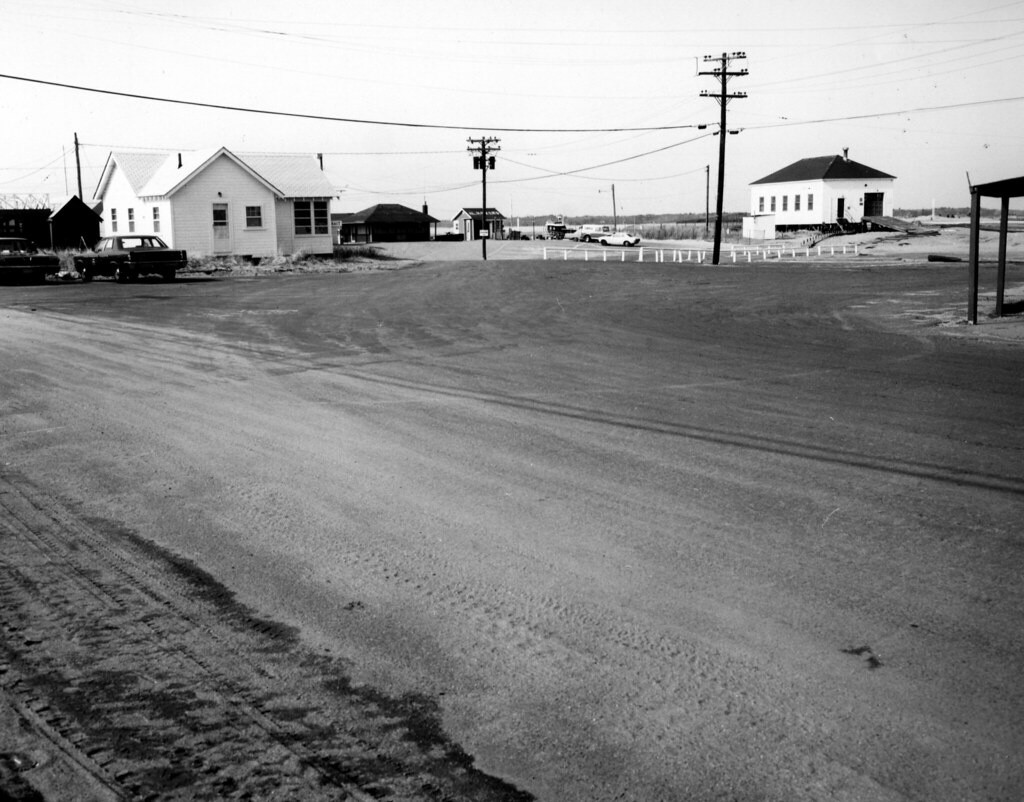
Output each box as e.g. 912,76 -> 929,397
1,238 -> 60,274
545,224 -> 640,247
73,235 -> 189,282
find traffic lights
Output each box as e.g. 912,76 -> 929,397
487,156 -> 495,169
